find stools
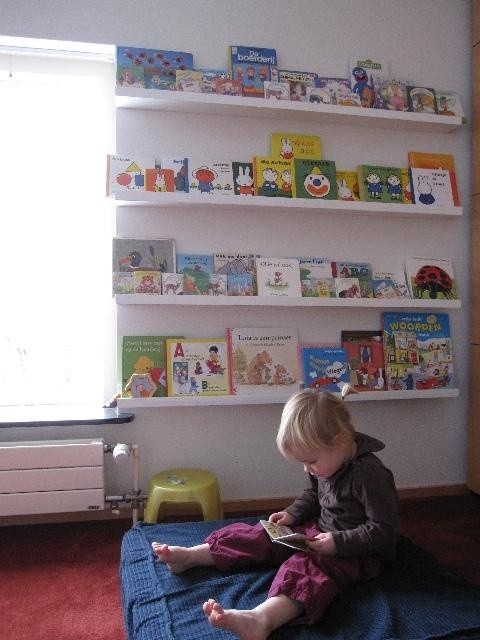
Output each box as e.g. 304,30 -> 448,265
143,467 -> 224,524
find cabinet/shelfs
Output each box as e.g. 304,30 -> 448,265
111,63 -> 465,411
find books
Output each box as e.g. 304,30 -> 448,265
341,329 -> 386,391
116,45 -> 242,96
348,57 -> 467,123
408,150 -> 460,206
259,520 -> 319,552
254,132 -> 337,198
255,257 -> 302,296
111,237 -> 259,297
225,327 -> 301,394
227,46 -> 361,108
106,154 -> 254,196
371,270 -> 412,300
335,164 -> 414,203
381,312 -> 455,390
166,339 -> 231,394
405,257 -> 457,300
121,336 -> 185,396
298,342 -> 350,392
290,256 -> 373,298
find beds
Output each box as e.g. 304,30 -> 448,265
117,511 -> 480,640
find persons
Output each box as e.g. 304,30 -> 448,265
206,346 -> 221,366
152,389 -> 399,639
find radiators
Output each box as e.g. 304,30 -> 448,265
1,438 -> 148,530
